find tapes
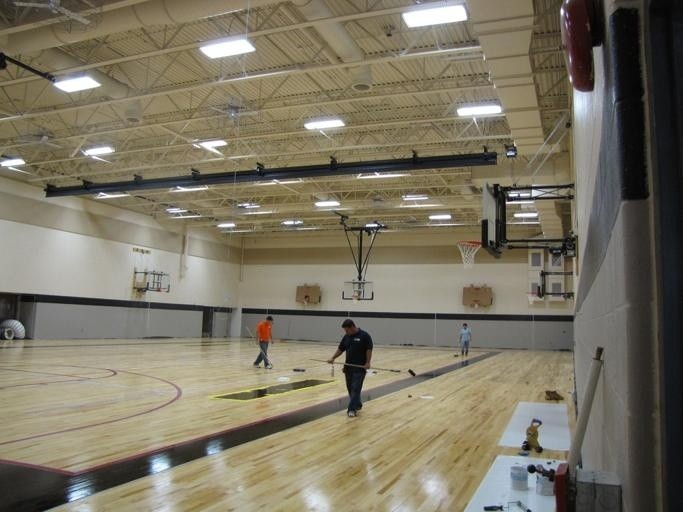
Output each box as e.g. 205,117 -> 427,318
510,466 -> 555,496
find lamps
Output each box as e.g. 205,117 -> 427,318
83,145 -> 115,156
304,116 -> 345,130
200,38 -> 256,59
53,74 -> 101,93
401,1 -> 468,28
1,157 -> 25,167
193,139 -> 228,150
504,143 -> 517,157
457,101 -> 503,118
43,158 -> 537,229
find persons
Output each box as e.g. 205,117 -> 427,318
327,318 -> 373,418
457,322 -> 472,358
250,315 -> 273,368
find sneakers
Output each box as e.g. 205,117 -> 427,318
253,363 -> 272,369
348,405 -> 362,417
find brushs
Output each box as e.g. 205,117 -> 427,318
485,500 -> 532,512
310,358 -> 416,376
